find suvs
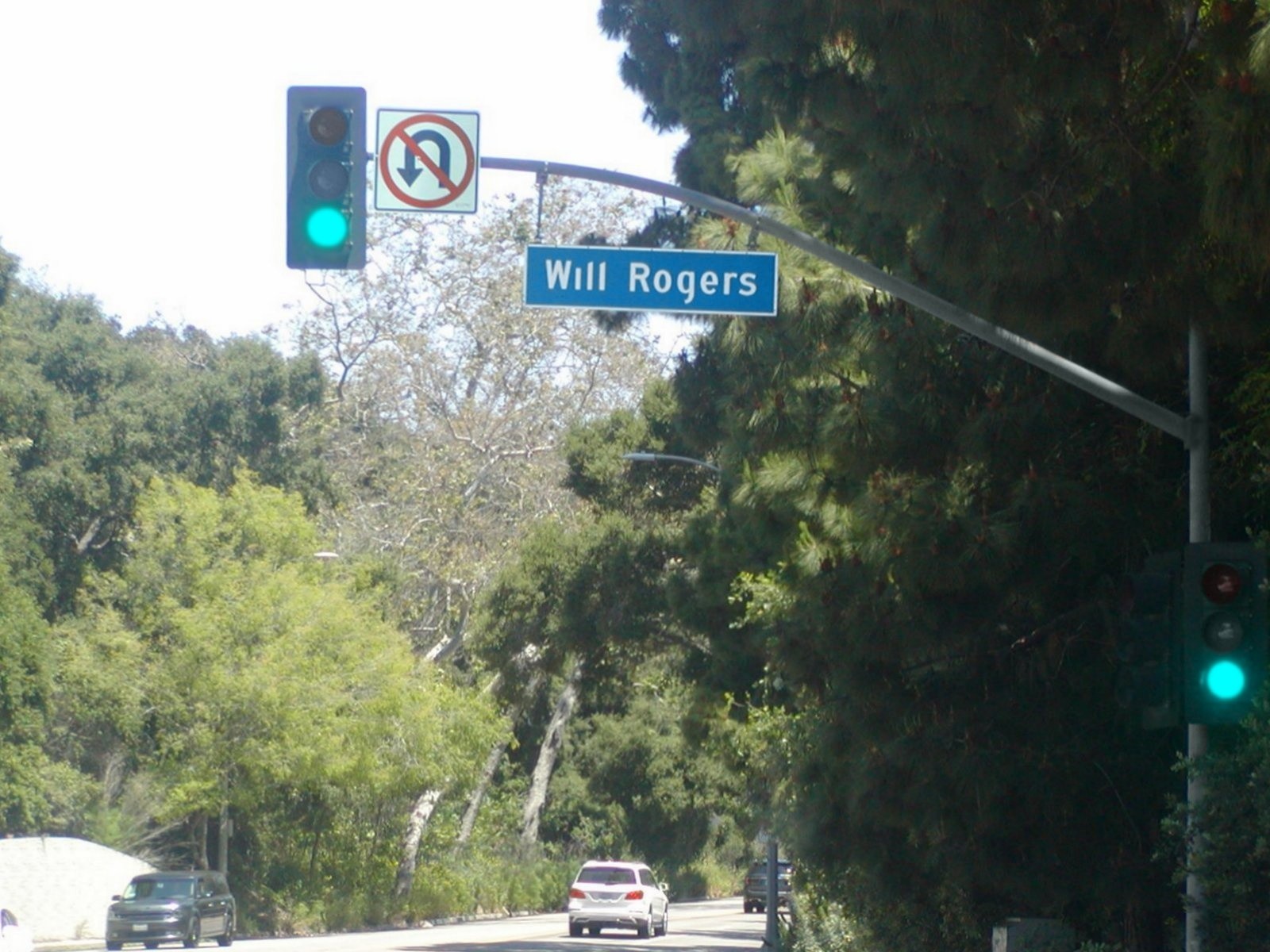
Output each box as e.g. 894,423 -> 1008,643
566,858 -> 669,938
741,861 -> 797,913
104,870 -> 237,950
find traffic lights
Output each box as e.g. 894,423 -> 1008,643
284,86 -> 368,270
1183,543 -> 1259,726
